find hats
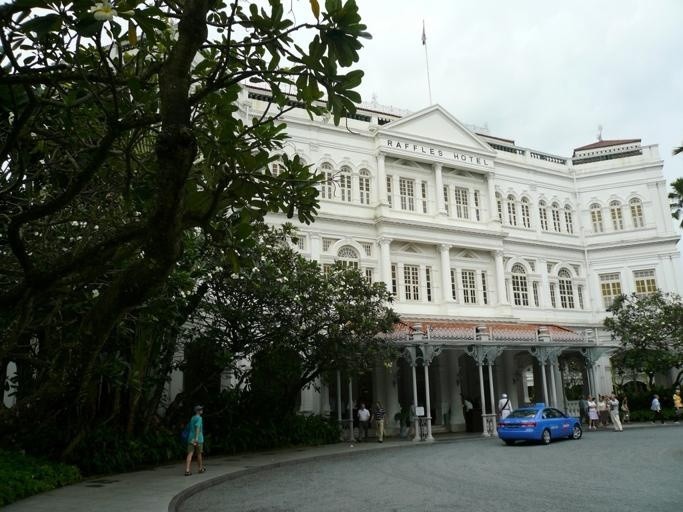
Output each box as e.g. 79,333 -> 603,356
194,405 -> 205,412
502,393 -> 507,398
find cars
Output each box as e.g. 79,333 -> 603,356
495,405 -> 582,447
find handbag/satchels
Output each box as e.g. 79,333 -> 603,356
181,423 -> 192,441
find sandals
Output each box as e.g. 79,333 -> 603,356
200,467 -> 207,473
184,471 -> 192,476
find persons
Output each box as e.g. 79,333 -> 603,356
369,399 -> 388,443
671,388 -> 683,424
184,405 -> 206,476
406,400 -> 419,438
498,393 -> 513,427
356,404 -> 370,442
650,393 -> 666,425
577,392 -> 632,432
